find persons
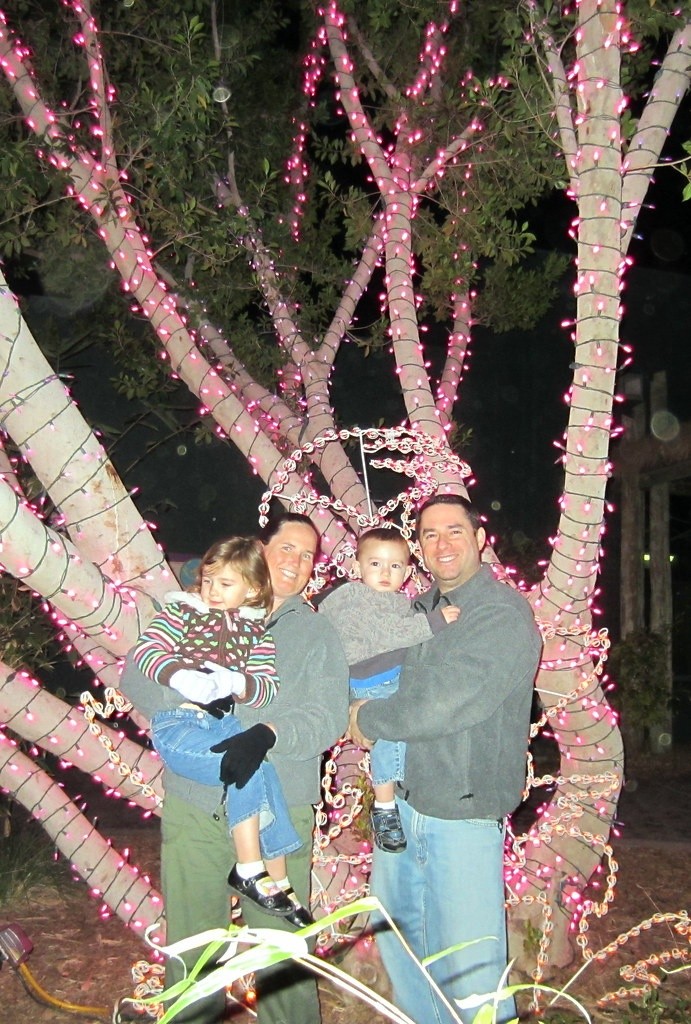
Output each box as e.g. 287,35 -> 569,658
118,511 -> 352,1024
319,528 -> 460,853
348,493 -> 545,1024
132,535 -> 319,929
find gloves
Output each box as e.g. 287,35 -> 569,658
170,668 -> 215,705
212,723 -> 275,790
203,661 -> 243,701
195,668 -> 235,720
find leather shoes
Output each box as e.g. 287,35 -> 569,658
227,865 -> 296,916
279,888 -> 317,930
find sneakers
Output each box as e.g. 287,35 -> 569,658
369,798 -> 407,853
